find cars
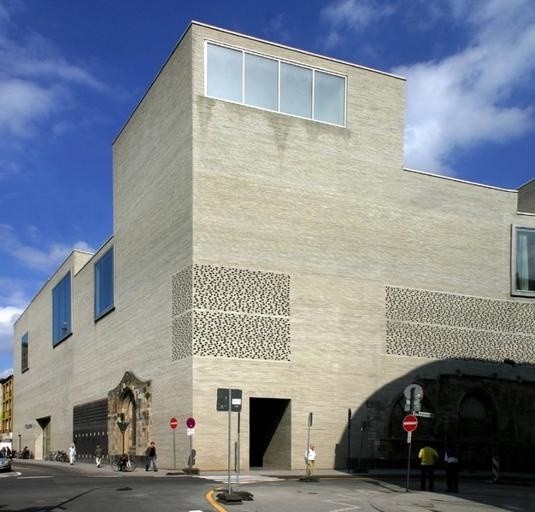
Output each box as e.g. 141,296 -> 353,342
0,450 -> 12,472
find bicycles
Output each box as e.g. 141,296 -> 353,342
43,450 -> 70,462
111,453 -> 138,472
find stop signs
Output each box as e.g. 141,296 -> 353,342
169,418 -> 178,428
402,415 -> 419,431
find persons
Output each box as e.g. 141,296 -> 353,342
442,442 -> 461,492
0,444 -> 30,460
417,440 -> 441,489
68,444 -> 76,465
305,443 -> 316,479
144,441 -> 158,472
94,444 -> 104,468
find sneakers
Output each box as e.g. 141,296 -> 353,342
98,464 -> 100,467
145,469 -> 158,472
70,463 -> 73,465
